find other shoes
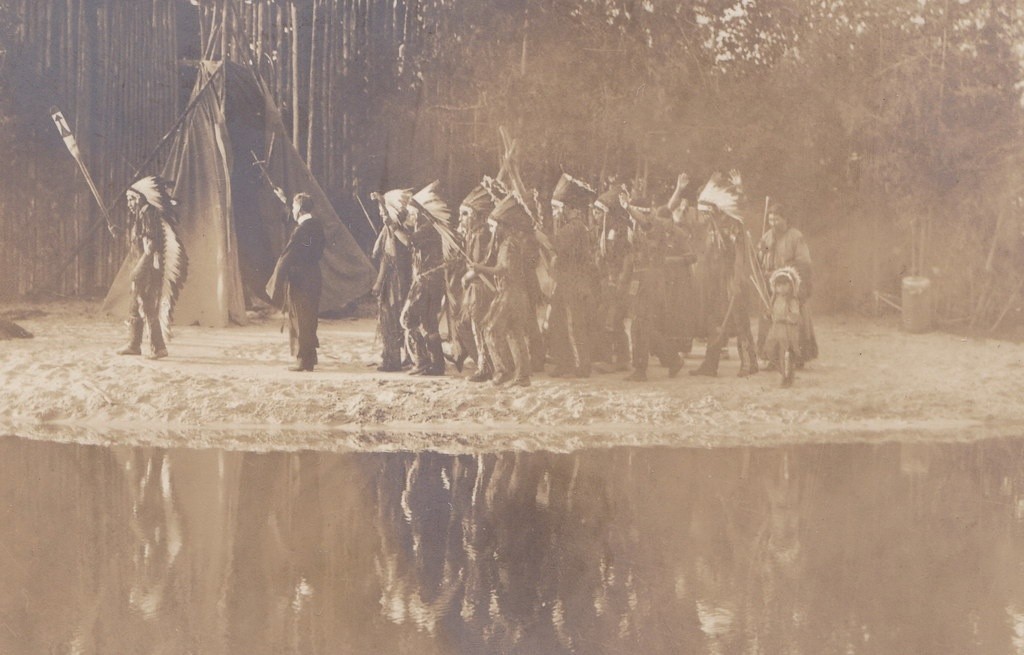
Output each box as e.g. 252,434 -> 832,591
373,346 -> 795,390
143,346 -> 168,359
288,358 -> 313,371
297,352 -> 317,365
117,344 -> 141,355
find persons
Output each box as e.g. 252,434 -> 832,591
372,168 -> 816,387
273,187 -> 326,372
108,188 -> 168,359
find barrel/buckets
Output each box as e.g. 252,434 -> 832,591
902,277 -> 932,333
